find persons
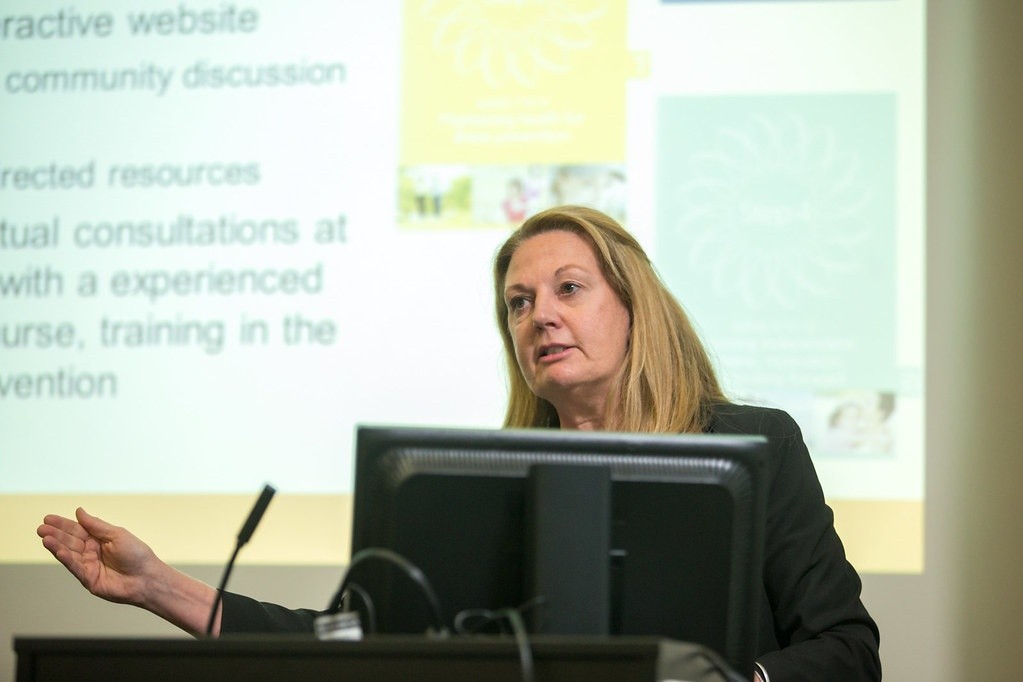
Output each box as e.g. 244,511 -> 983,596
37,207 -> 882,682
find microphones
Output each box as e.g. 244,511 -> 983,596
207,485 -> 275,636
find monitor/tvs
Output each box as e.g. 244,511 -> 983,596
349,427 -> 769,682
